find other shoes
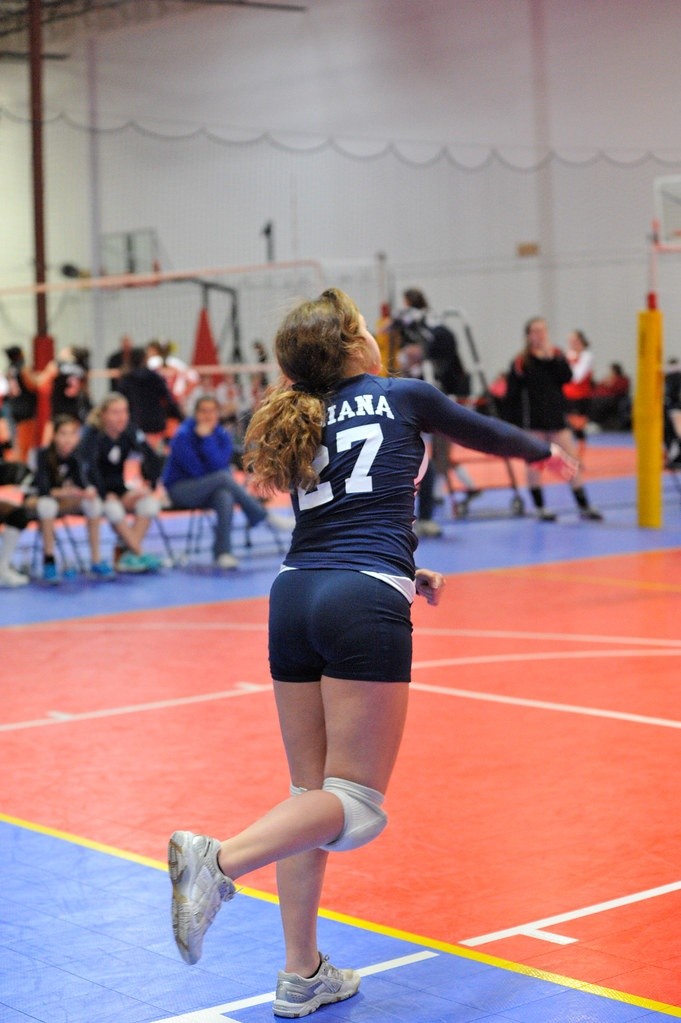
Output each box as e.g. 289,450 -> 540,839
213,553 -> 240,571
579,507 -> 602,519
0,567 -> 29,587
537,506 -> 555,520
415,520 -> 443,537
450,487 -> 481,501
266,515 -> 298,532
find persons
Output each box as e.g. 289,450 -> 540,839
503,318 -> 605,522
664,360 -> 681,474
254,342 -> 268,390
390,289 -> 510,539
162,396 -> 295,571
563,329 -> 632,441
1,336 -> 202,587
168,288 -> 580,1019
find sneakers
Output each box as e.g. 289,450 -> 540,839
166,831 -> 242,966
272,951 -> 362,1018
88,560 -> 115,582
118,550 -> 147,573
139,554 -> 171,577
43,564 -> 61,588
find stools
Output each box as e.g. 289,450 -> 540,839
29,484 -> 211,581
